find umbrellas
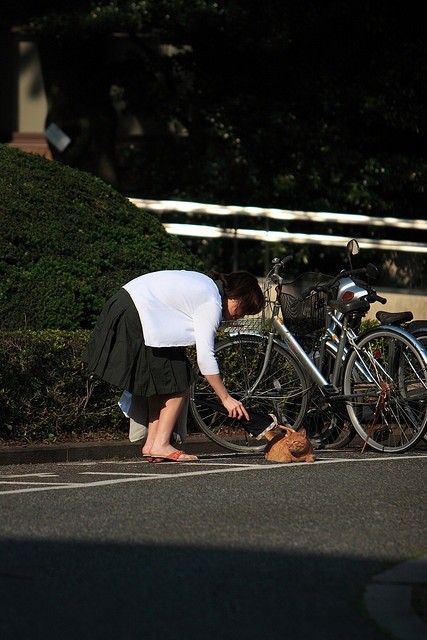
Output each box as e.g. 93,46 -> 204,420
191,398 -> 296,441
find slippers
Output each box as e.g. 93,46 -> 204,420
142,450 -> 200,462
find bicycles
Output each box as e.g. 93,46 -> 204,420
268,268 -> 426,449
189,255 -> 426,453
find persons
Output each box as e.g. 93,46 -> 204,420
80,270 -> 268,464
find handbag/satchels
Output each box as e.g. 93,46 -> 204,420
128,387 -> 189,445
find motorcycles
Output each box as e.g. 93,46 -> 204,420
283,239 -> 426,443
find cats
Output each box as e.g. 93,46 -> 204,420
265,429 -> 317,465
